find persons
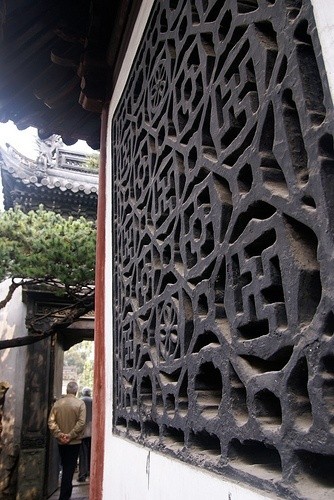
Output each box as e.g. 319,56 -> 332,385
75,385 -> 93,482
47,381 -> 86,499
